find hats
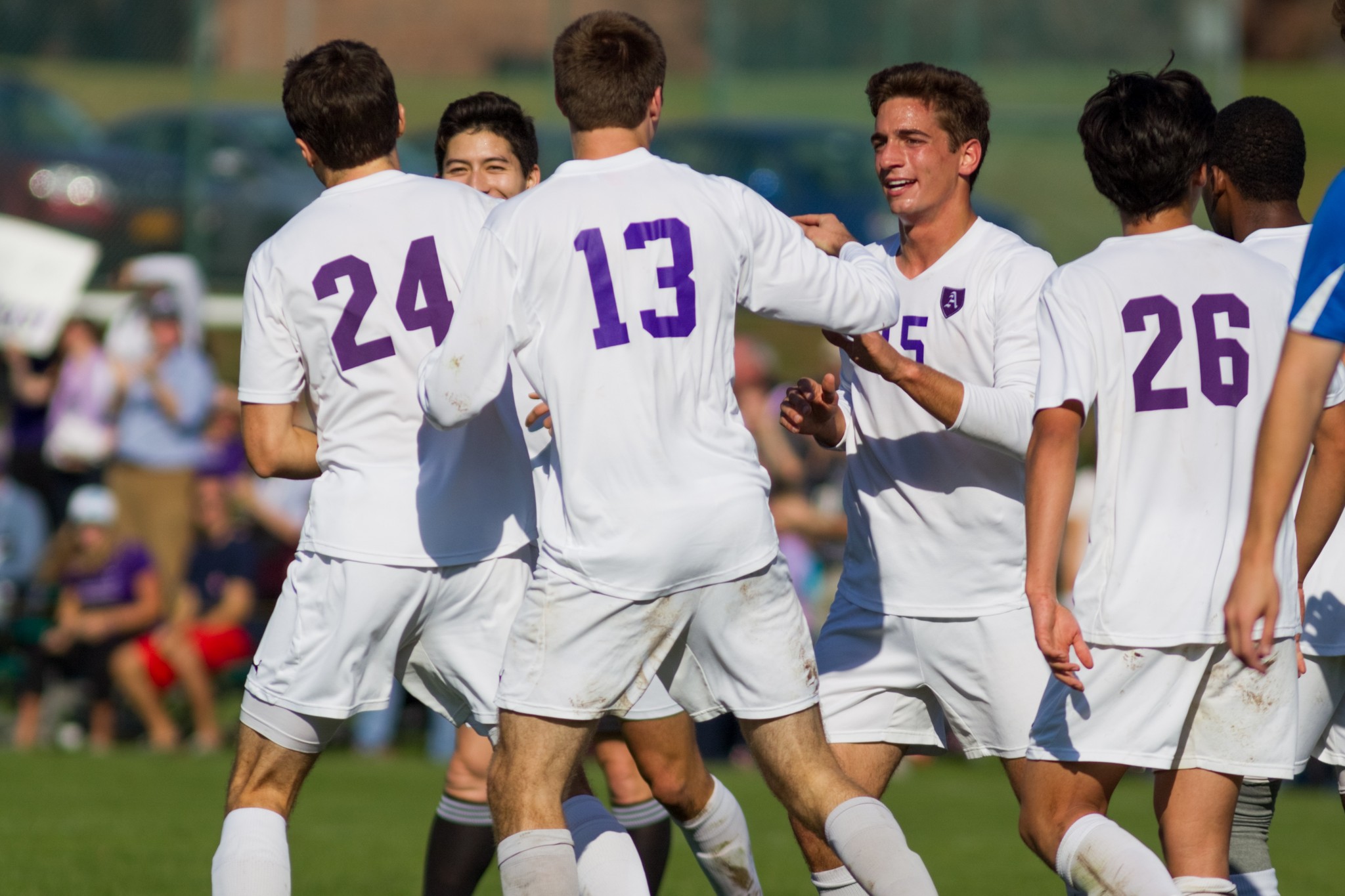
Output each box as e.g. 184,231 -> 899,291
64,485 -> 119,523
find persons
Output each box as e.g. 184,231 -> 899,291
412,7 -> 943,895
2,40 -> 1345,896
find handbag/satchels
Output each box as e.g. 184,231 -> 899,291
41,417 -> 112,470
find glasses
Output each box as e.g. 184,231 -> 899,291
150,311 -> 178,322
